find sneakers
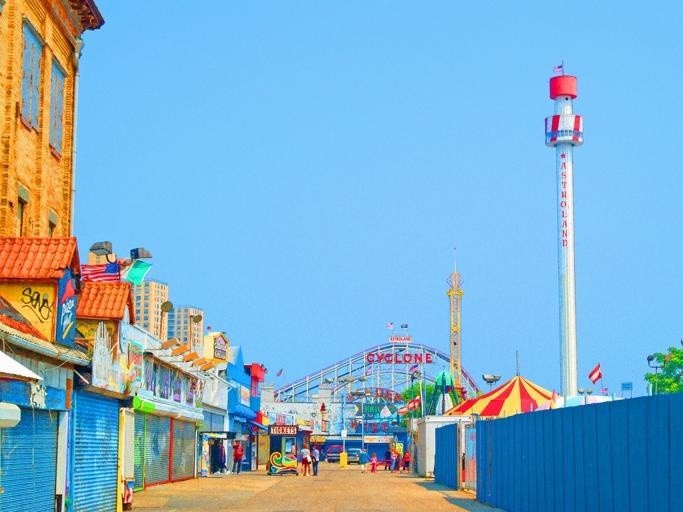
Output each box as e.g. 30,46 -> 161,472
214,469 -> 240,475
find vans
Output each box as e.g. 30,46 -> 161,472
327,444 -> 343,463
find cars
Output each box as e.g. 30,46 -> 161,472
347,448 -> 362,464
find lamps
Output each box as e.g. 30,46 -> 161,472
145,302 -> 215,372
91,242 -> 151,281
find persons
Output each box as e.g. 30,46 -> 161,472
359,448 -> 412,473
312,444 -> 319,476
217,442 -> 228,473
232,440 -> 244,475
301,443 -> 311,475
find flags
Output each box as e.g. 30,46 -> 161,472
387,322 -> 394,327
81,262 -> 119,281
600,388 -> 609,393
119,257 -> 154,287
589,364 -> 603,384
553,65 -> 562,72
